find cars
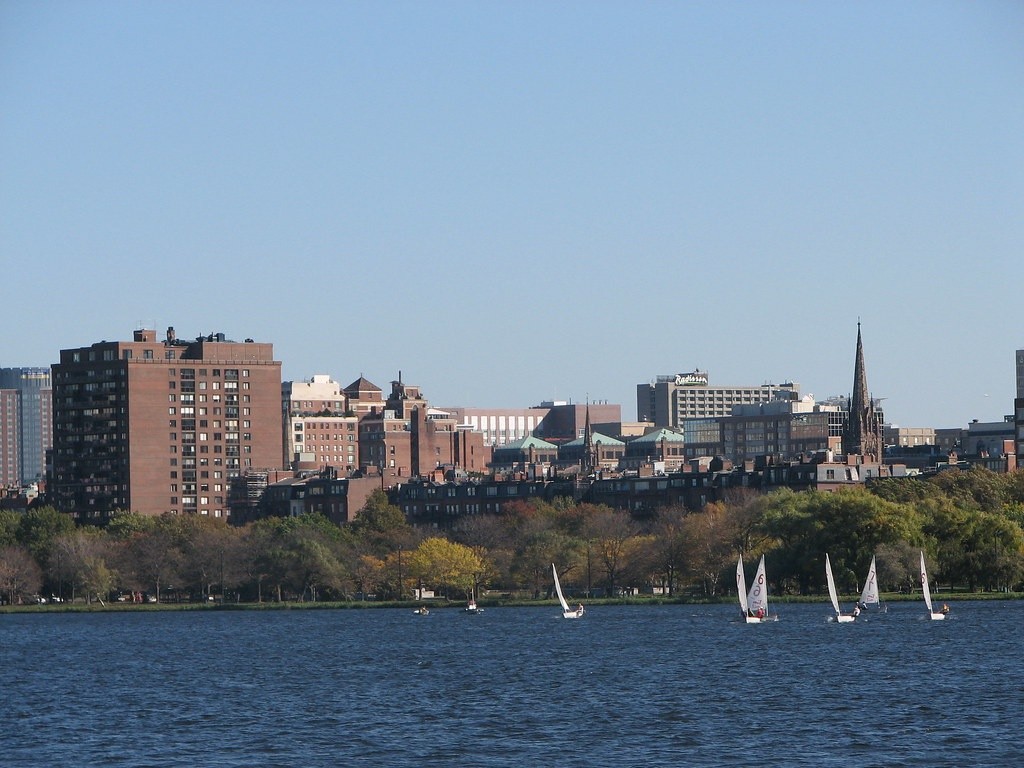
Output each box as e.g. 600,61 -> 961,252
37,594 -> 63,603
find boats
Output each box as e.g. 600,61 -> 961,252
464,588 -> 485,615
413,610 -> 430,616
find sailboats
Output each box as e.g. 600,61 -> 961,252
732,553 -> 778,624
853,554 -> 887,617
825,552 -> 856,623
920,550 -> 945,621
552,563 -> 582,619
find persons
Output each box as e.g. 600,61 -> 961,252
746,608 -> 755,617
421,604 -> 426,613
861,602 -> 868,611
854,602 -> 861,617
939,604 -> 950,615
757,607 -> 765,618
578,603 -> 583,610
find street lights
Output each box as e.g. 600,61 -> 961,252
219,549 -> 224,603
398,544 -> 402,601
